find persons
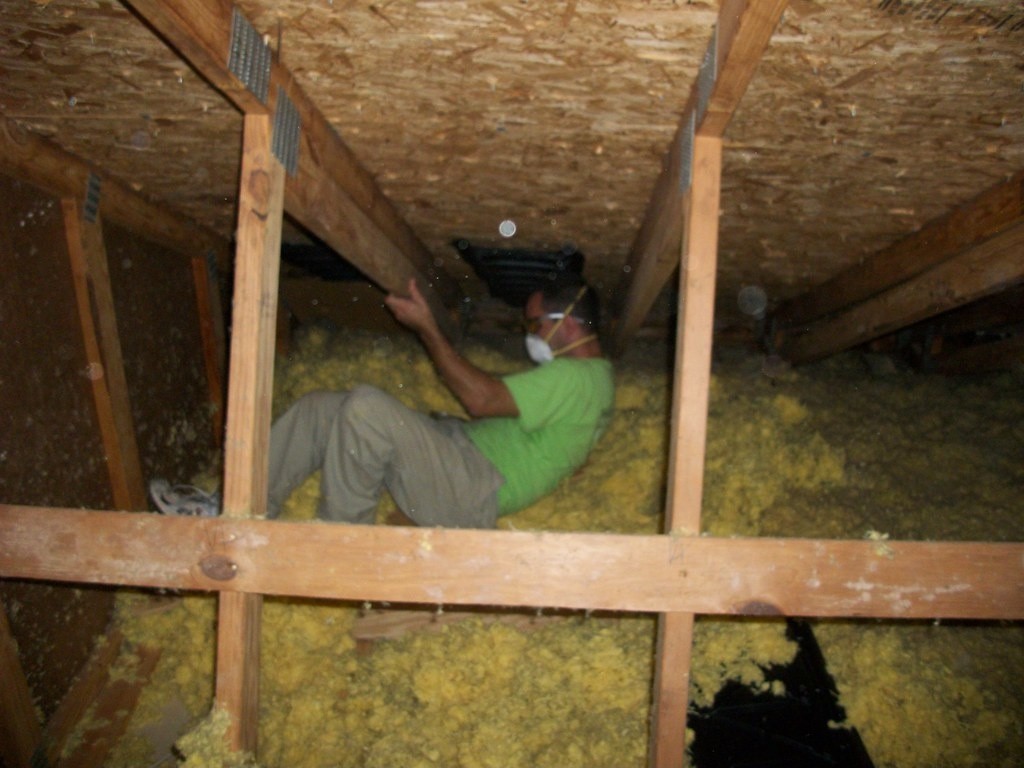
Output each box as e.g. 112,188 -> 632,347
150,277 -> 615,527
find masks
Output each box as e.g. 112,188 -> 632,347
525,333 -> 555,364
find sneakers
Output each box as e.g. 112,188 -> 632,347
150,479 -> 221,515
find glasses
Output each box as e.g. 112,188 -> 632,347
525,313 -> 586,334
